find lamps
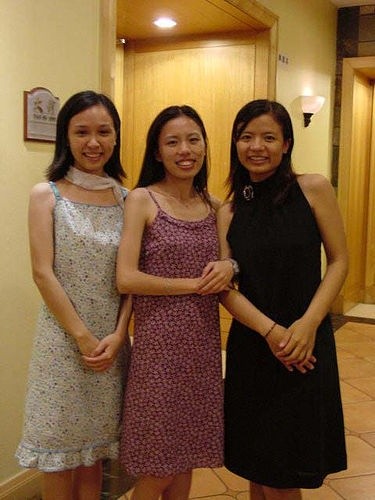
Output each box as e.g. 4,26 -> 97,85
301,95 -> 325,126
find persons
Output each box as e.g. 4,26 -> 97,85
15,90 -> 130,500
214,99 -> 351,500
117,106 -> 237,500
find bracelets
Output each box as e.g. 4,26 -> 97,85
263,322 -> 277,339
222,257 -> 239,273
